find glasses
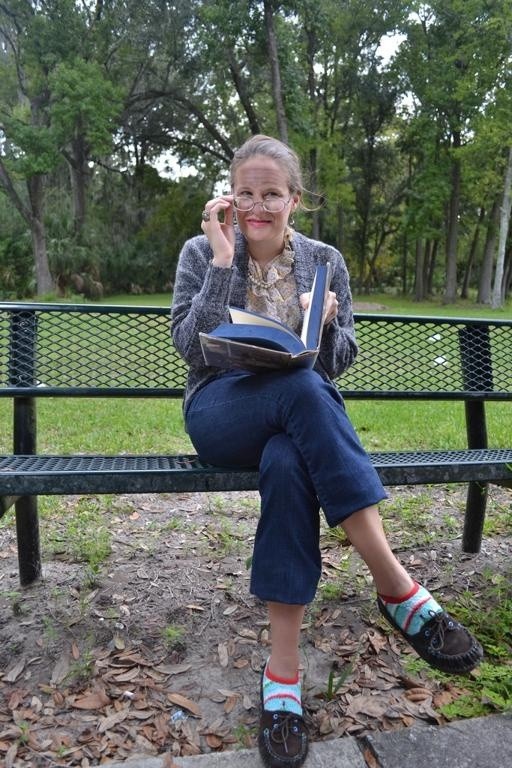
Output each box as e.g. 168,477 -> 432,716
229,194 -> 294,213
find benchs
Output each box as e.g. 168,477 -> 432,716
0,297 -> 511,588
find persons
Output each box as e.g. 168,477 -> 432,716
169,133 -> 483,768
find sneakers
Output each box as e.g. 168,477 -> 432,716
377,578 -> 484,677
257,657 -> 309,766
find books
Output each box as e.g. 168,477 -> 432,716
198,261 -> 331,374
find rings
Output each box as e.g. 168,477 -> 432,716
201,211 -> 210,222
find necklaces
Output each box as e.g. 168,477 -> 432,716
247,269 -> 279,289
